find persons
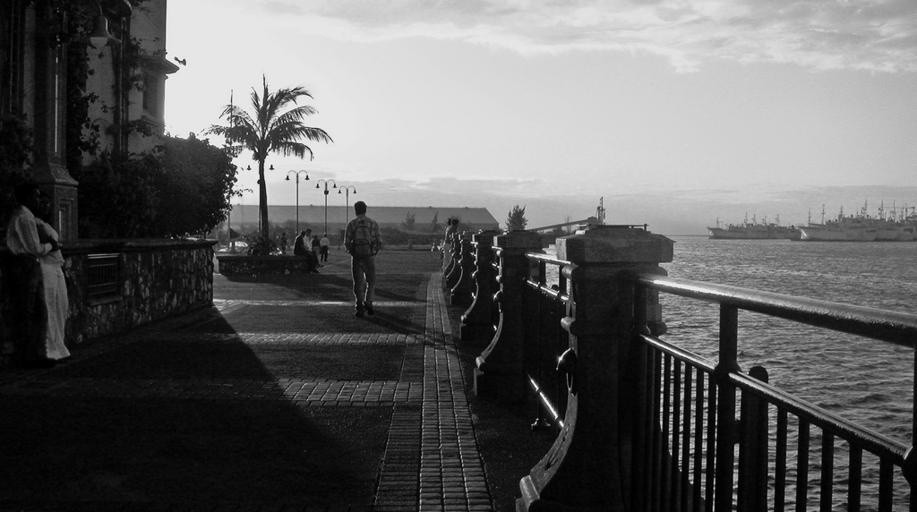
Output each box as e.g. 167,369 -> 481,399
311,235 -> 319,257
443,218 -> 452,236
343,201 -> 383,318
4,181 -> 60,367
319,232 -> 330,262
293,230 -> 319,274
30,190 -> 72,363
280,232 -> 288,255
442,219 -> 459,279
302,229 -> 324,268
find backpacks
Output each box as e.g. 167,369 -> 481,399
348,220 -> 373,258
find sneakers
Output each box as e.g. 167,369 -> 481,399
365,300 -> 373,315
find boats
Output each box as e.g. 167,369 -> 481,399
706,199 -> 917,244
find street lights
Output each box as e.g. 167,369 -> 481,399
315,178 -> 337,234
246,162 -> 276,232
338,185 -> 357,225
284,169 -> 311,236
